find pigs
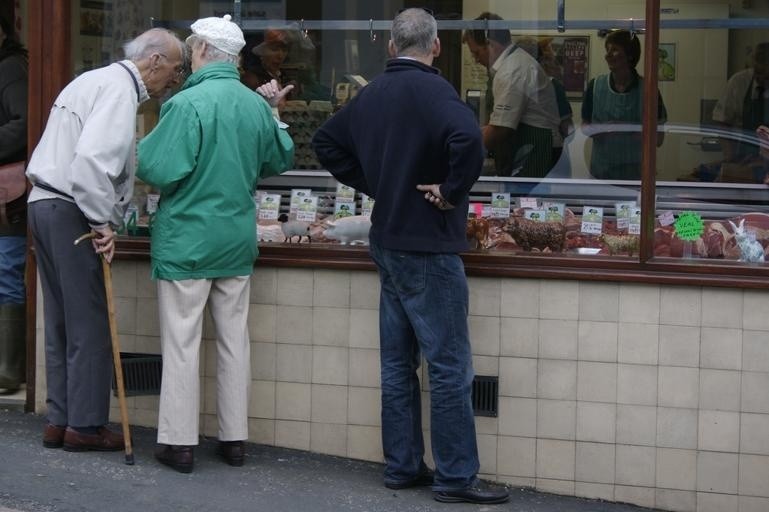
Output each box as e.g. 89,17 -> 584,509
322,216 -> 372,247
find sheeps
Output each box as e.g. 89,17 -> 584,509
596,232 -> 640,257
277,214 -> 312,243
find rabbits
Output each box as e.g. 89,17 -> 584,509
728,218 -> 765,263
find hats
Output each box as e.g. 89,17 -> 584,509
185,14 -> 247,57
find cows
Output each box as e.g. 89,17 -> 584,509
501,216 -> 568,253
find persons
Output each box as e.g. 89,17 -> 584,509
21,26 -> 185,453
517,36 -> 576,145
310,5 -> 512,502
0,2 -> 26,405
711,42 -> 769,183
135,15 -> 297,470
242,30 -> 294,91
578,28 -> 667,182
462,13 -> 572,177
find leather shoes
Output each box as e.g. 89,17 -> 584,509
431,478 -> 510,504
214,441 -> 246,467
63,426 -> 134,452
384,464 -> 435,489
155,444 -> 196,474
42,424 -> 66,448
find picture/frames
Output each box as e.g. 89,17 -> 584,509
511,32 -> 590,100
657,41 -> 678,84
